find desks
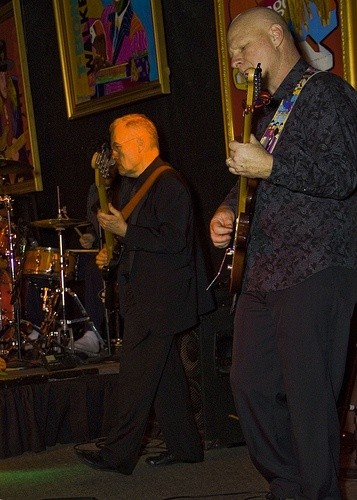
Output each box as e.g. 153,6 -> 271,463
0,360 -> 124,390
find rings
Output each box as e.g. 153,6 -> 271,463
228,158 -> 232,168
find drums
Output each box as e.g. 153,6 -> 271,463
0,268 -> 33,341
23,247 -> 80,280
0,215 -> 18,257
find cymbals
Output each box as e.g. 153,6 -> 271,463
30,215 -> 90,229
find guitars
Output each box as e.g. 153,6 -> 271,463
222,62 -> 273,305
90,141 -> 125,315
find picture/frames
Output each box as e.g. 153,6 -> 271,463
214,0 -> 357,159
0,0 -> 43,196
53,0 -> 171,121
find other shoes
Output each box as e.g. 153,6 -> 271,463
77,449 -> 121,472
68,331 -> 101,353
147,450 -> 182,466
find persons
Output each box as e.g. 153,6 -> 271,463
74,113 -> 215,475
209,7 -> 357,500
